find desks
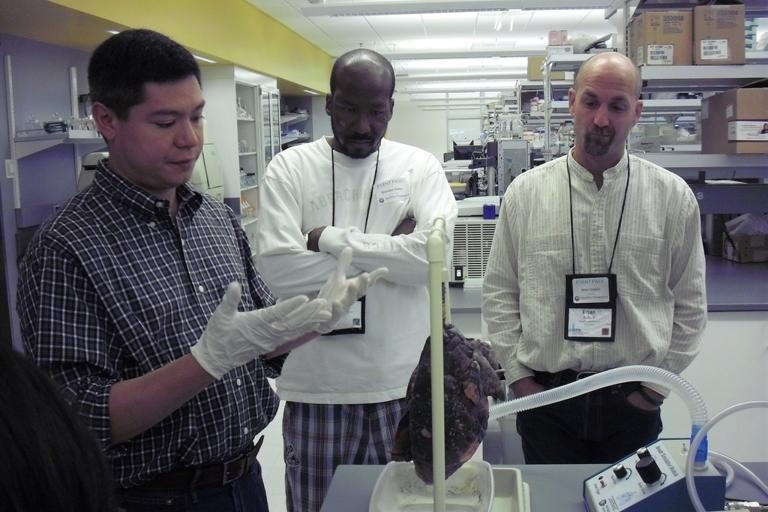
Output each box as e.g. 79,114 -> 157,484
315,462 -> 767,512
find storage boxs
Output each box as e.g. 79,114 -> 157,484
629,5 -> 691,66
693,2 -> 745,65
700,213 -> 742,256
721,234 -> 767,264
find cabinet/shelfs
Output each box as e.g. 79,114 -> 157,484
280,113 -> 311,152
262,84 -> 282,168
484,53 -> 768,188
235,81 -> 264,258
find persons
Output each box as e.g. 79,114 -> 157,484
256,48 -> 460,512
16,28 -> 391,512
1,341 -> 113,512
482,47 -> 709,465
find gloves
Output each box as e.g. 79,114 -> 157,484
190,282 -> 332,380
313,246 -> 387,333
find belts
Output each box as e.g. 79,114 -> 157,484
533,370 -> 633,396
149,435 -> 263,491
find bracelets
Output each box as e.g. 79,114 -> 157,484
638,388 -> 668,412
